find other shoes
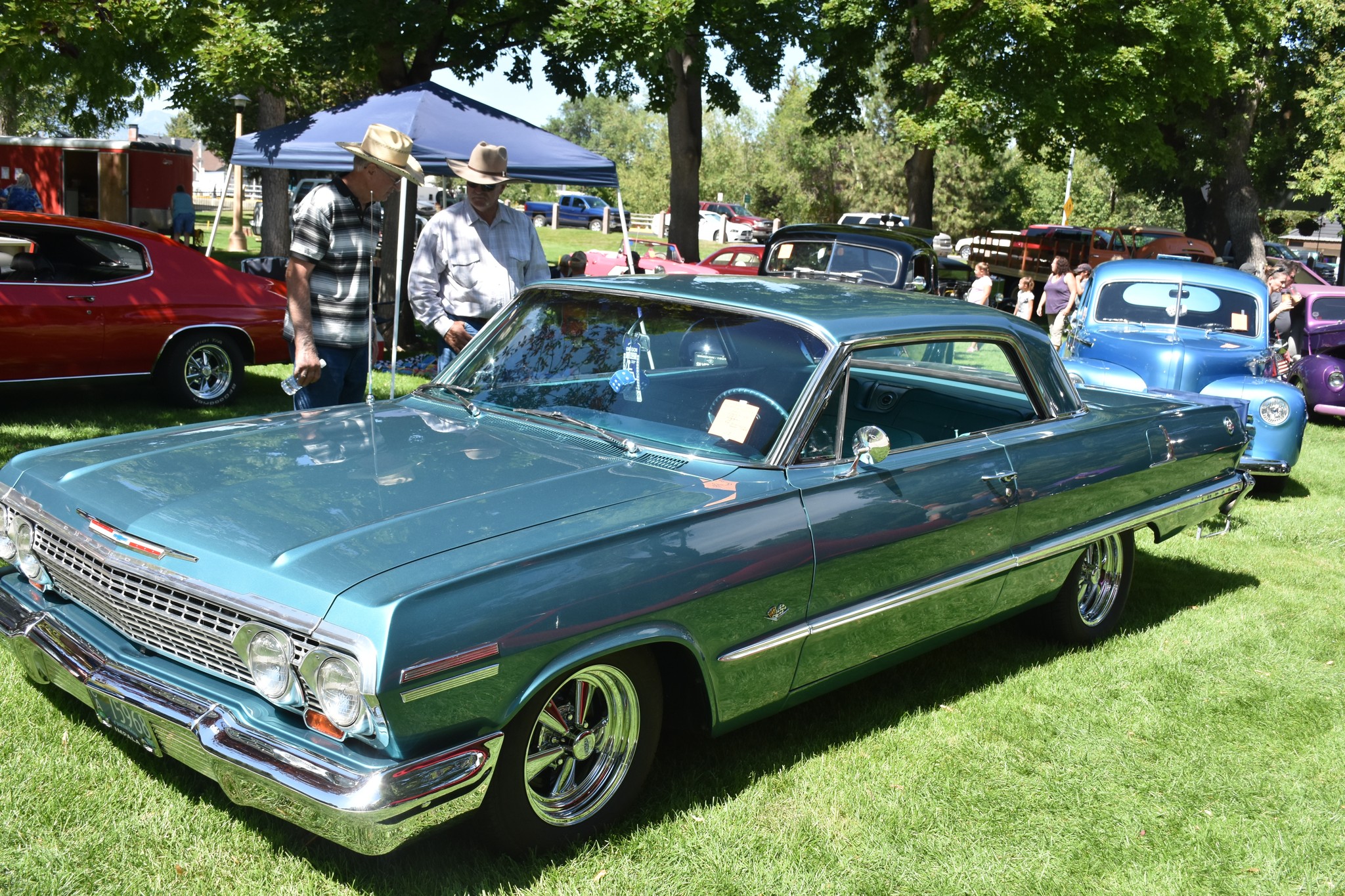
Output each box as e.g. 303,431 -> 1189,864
967,346 -> 979,352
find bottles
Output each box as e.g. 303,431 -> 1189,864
281,358 -> 326,396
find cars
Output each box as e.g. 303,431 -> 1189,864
546,213 -> 1345,427
1,208 -> 292,408
651,210 -> 753,243
247,171 -> 506,255
1,263 -> 1259,857
1057,254 -> 1309,498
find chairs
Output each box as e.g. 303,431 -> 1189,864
6,252 -> 38,283
615,377 -> 707,430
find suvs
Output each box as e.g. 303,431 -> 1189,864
666,200 -> 774,245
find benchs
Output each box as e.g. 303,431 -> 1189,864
51,262 -> 144,287
795,370 -> 1026,459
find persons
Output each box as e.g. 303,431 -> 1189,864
283,122 -> 424,412
1265,266 -> 1294,381
1270,260 -> 1303,366
568,250 -> 588,277
965,261 -> 992,352
1013,276 -> 1034,321
1070,264 -> 1092,330
169,185 -> 196,247
0,172 -> 44,212
1238,262 -> 1261,276
1036,256 -> 1076,351
623,251 -> 646,274
1213,256 -> 1229,266
550,254 -> 572,280
407,140 -> 553,377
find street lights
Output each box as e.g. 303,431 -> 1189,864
228,92 -> 253,252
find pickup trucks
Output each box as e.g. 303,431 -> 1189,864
523,194 -> 632,233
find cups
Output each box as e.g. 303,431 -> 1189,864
1281,292 -> 1291,303
1286,286 -> 1301,302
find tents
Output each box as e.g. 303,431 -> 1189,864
205,80 -> 656,402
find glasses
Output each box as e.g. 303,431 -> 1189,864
466,181 -> 504,192
1288,274 -> 1295,279
1267,268 -> 1285,279
559,264 -> 569,268
364,164 -> 401,184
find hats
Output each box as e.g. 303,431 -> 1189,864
1239,263 -> 1260,274
571,251 -> 588,265
445,141 -> 531,185
1073,263 -> 1092,274
1213,257 -> 1228,266
335,122 -> 425,187
